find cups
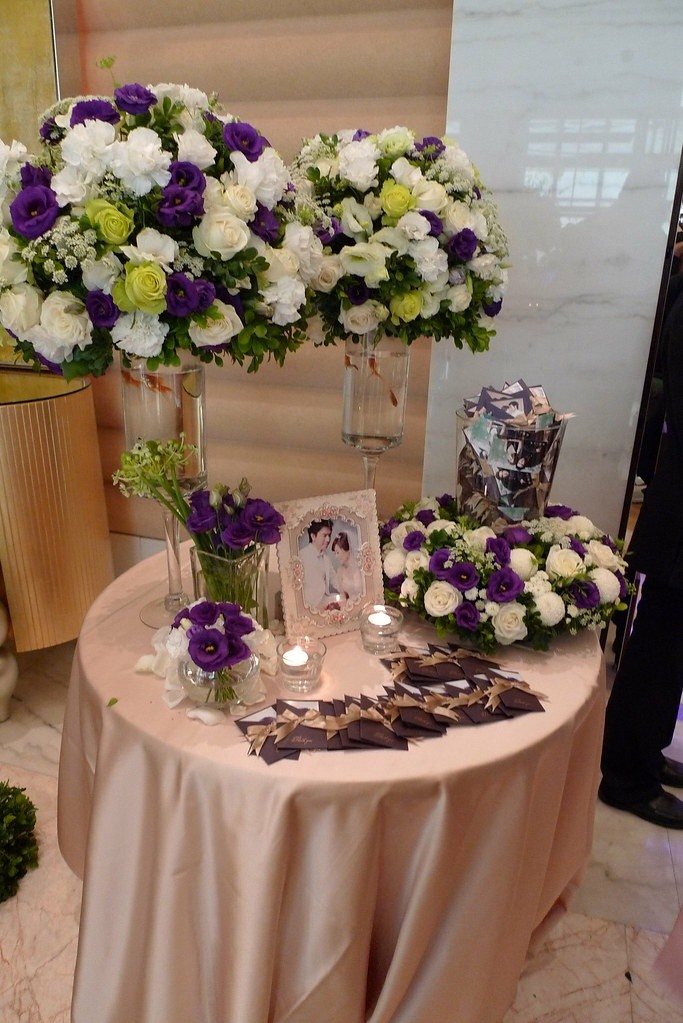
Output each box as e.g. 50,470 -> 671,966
453,408 -> 567,525
359,605 -> 403,655
276,636 -> 327,693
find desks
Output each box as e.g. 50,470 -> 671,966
55,522 -> 607,1023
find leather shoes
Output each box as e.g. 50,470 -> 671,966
598,777 -> 683,832
658,757 -> 683,788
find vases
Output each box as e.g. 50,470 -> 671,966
109,348 -> 211,630
186,542 -> 272,631
339,327 -> 413,490
177,647 -> 262,710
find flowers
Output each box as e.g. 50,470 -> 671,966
376,495 -> 638,655
280,129 -> 505,354
117,437 -> 288,558
136,594 -> 279,711
2,85 -> 327,381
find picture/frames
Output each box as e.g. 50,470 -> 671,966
265,485 -> 389,647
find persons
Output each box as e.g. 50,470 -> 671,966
300,521 -> 363,612
597,224 -> 683,830
